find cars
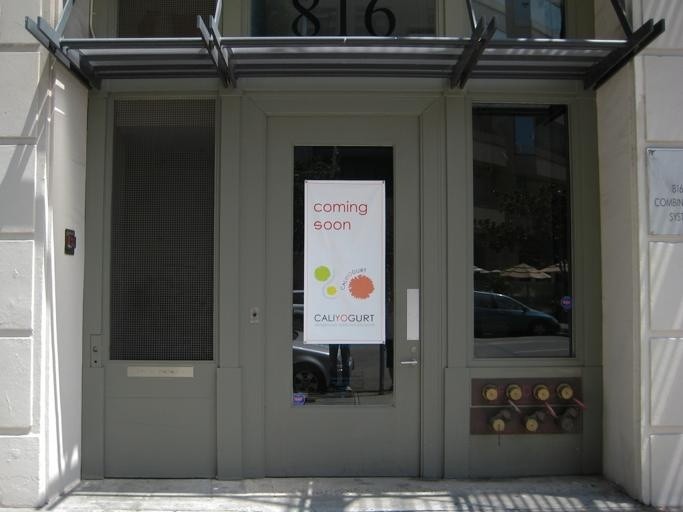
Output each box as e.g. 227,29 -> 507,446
290,285 -> 561,403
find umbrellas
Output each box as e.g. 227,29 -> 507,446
498,261 -> 551,283
536,261 -> 570,279
474,264 -> 499,287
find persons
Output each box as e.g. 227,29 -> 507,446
385,338 -> 394,391
325,344 -> 354,393
